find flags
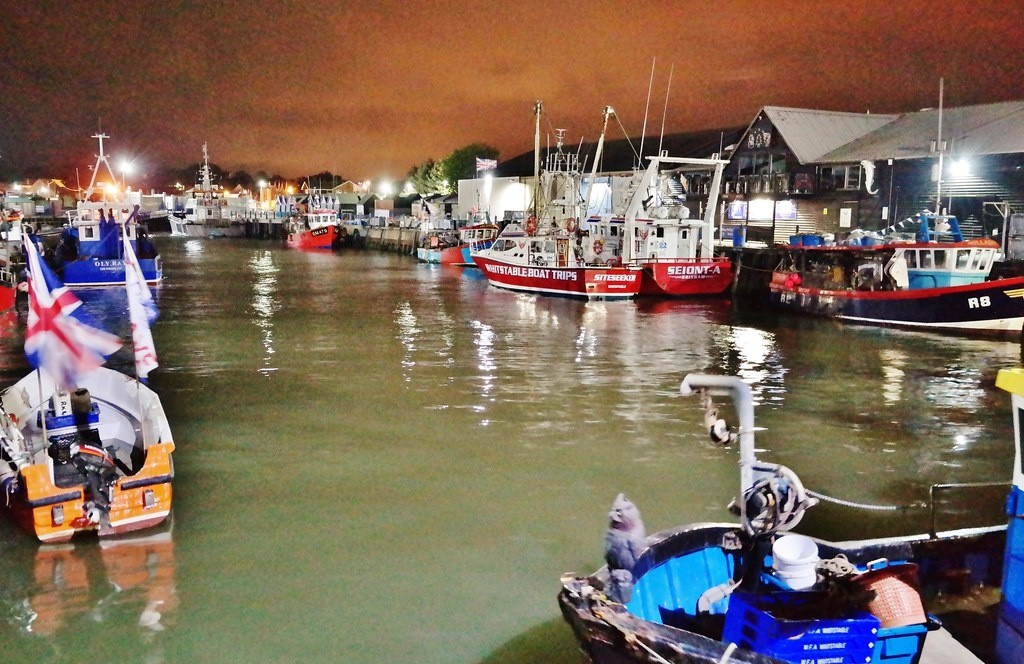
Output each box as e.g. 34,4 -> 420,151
122,223 -> 158,383
477,158 -> 497,171
23,227 -> 122,388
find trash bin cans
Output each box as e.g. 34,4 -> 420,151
789,233 -> 824,246
861,236 -> 885,246
732,226 -> 747,249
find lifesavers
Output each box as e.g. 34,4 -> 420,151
527,215 -> 536,236
567,218 -> 576,232
594,241 -> 603,255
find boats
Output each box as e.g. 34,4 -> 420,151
0,216 -> 70,241
72,118 -> 168,232
417,232 -> 496,265
558,367 -> 1023,664
735,155 -> 1024,339
470,102 -> 643,300
0,224 -> 176,542
287,225 -> 347,253
585,105 -> 733,298
168,141 -> 245,236
